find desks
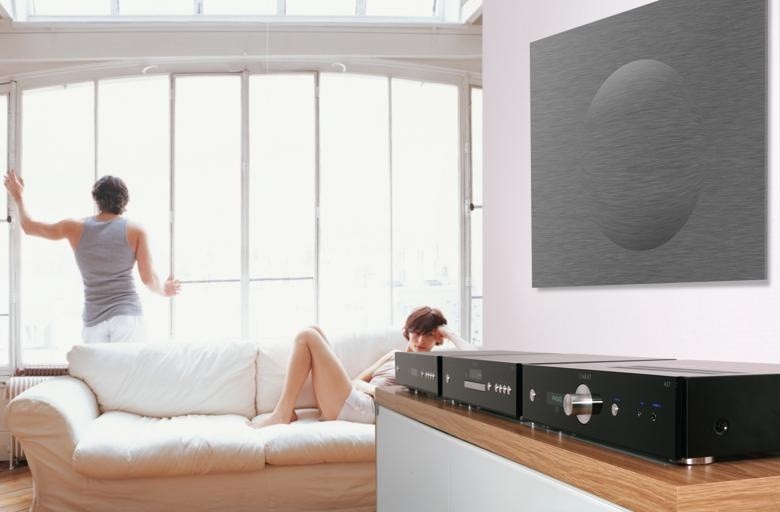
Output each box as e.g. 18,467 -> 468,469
375,386 -> 780,511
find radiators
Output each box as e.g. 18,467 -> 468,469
4,376 -> 69,469
13,366 -> 68,375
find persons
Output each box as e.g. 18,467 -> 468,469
246,305 -> 479,429
2,167 -> 183,344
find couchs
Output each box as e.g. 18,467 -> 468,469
6,335 -> 408,511
